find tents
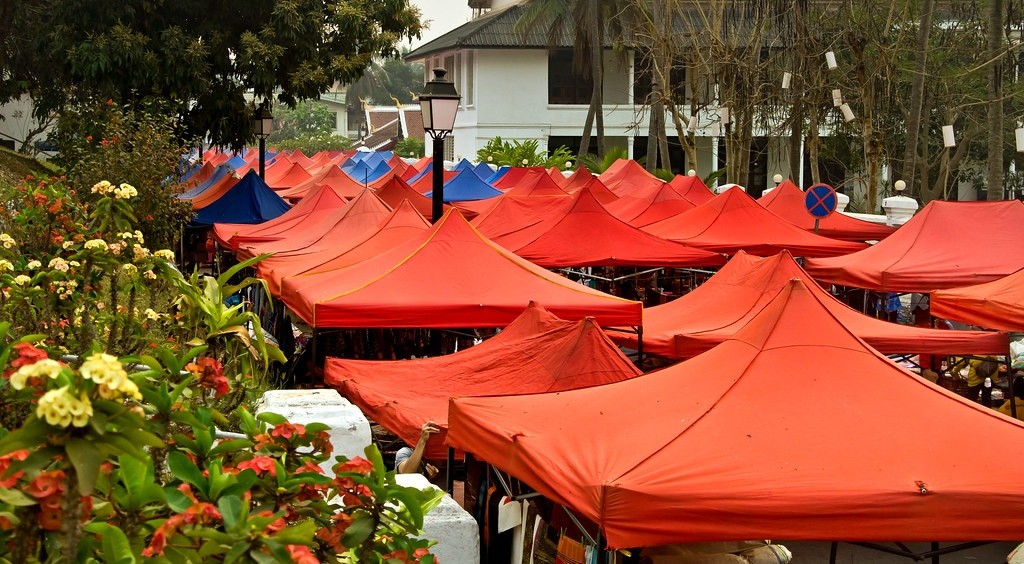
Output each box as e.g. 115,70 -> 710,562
164,147 -> 1024,564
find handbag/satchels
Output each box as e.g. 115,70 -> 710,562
976,356 -> 998,379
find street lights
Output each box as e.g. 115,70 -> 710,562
253,103 -> 277,186
419,67 -> 462,226
360,126 -> 367,145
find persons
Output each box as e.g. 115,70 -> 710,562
395,421 -> 441,487
948,354 -> 1000,407
998,376 -> 1024,421
